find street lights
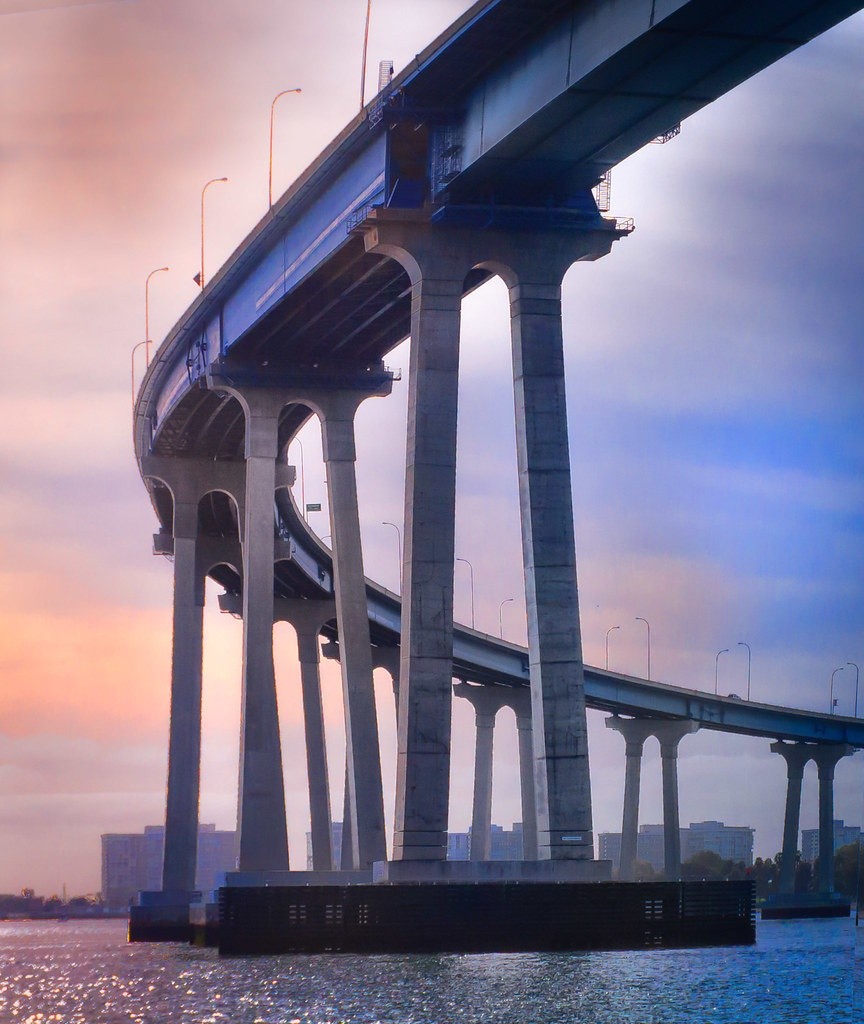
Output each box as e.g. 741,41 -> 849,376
382,523 -> 402,595
202,178 -> 228,290
457,558 -> 474,630
132,340 -> 152,408
146,267 -> 169,368
606,626 -> 620,670
715,649 -> 729,696
636,618 -> 650,681
269,88 -> 302,210
500,598 -> 513,640
737,643 -> 750,701
846,663 -> 859,718
830,667 -> 844,715
